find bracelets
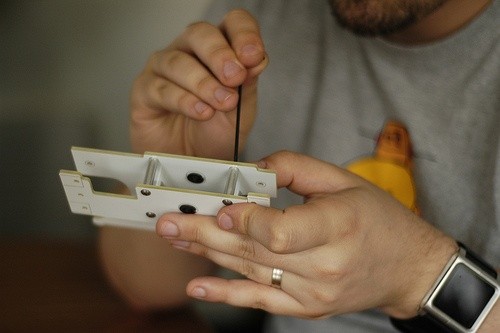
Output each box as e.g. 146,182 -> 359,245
388,239 -> 500,333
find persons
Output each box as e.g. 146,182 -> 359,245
93,0 -> 500,333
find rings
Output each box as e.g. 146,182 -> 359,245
270,268 -> 284,291
